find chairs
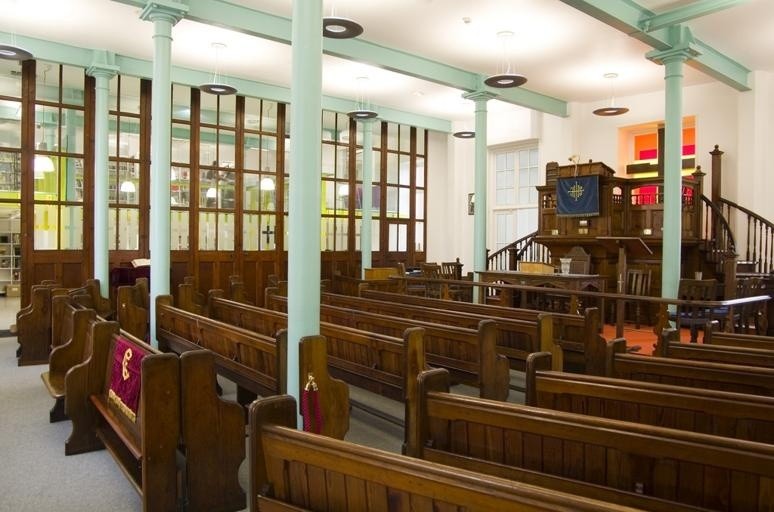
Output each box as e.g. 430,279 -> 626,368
395,260 -> 470,302
609,268 -> 653,329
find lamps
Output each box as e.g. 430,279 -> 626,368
206,178 -> 220,198
35,65 -> 55,171
197,42 -> 238,95
120,116 -> 136,193
593,72 -> 629,117
344,76 -> 378,122
340,183 -> 352,195
454,113 -> 476,139
261,100 -> 274,191
483,29 -> 526,88
323,0 -> 364,39
34,171 -> 44,179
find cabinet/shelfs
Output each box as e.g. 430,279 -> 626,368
0,216 -> 21,295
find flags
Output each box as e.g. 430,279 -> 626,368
106,336 -> 147,423
555,175 -> 600,217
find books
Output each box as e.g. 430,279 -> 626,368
131,258 -> 150,268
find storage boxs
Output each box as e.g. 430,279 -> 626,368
5,285 -> 21,297
